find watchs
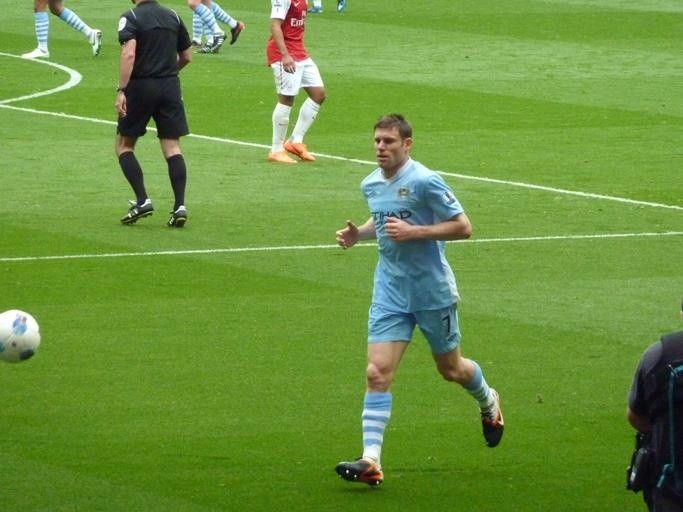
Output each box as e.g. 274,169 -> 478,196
116,86 -> 126,92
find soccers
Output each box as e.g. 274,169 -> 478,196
0,308 -> 40,362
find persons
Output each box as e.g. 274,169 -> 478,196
266,0 -> 327,164
628,305 -> 683,511
20,1 -> 106,59
307,0 -> 347,13
336,113 -> 506,485
188,0 -> 227,55
190,0 -> 244,52
114,0 -> 193,229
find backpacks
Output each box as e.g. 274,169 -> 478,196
640,332 -> 683,501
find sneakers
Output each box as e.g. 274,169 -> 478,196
21,47 -> 50,60
229,20 -> 245,46
120,198 -> 155,225
167,204 -> 188,228
337,0 -> 345,11
268,149 -> 298,165
478,387 -> 506,448
283,139 -> 317,163
307,6 -> 323,13
190,32 -> 227,53
332,455 -> 383,488
88,28 -> 103,56
